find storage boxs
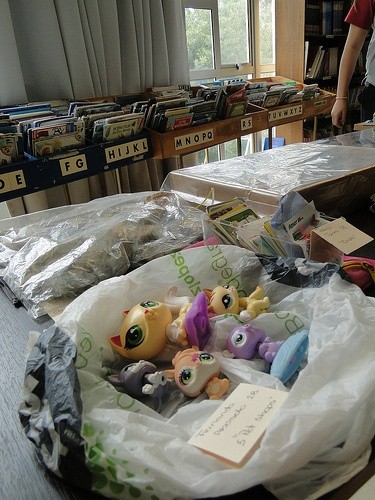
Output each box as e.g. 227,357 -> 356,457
121,88 -> 270,160
37,95 -> 154,188
223,75 -> 337,128
0,152 -> 47,202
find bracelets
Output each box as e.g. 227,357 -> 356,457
335,96 -> 348,100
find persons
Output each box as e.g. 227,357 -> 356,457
331,0 -> 375,129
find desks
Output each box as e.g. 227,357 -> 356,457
0,192 -> 179,500
177,128 -> 375,224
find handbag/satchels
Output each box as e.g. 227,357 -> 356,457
23,246 -> 375,496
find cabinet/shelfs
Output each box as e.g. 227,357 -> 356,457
276,0 -> 365,146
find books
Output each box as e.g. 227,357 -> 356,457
304,0 -> 366,143
0,75 -> 332,177
